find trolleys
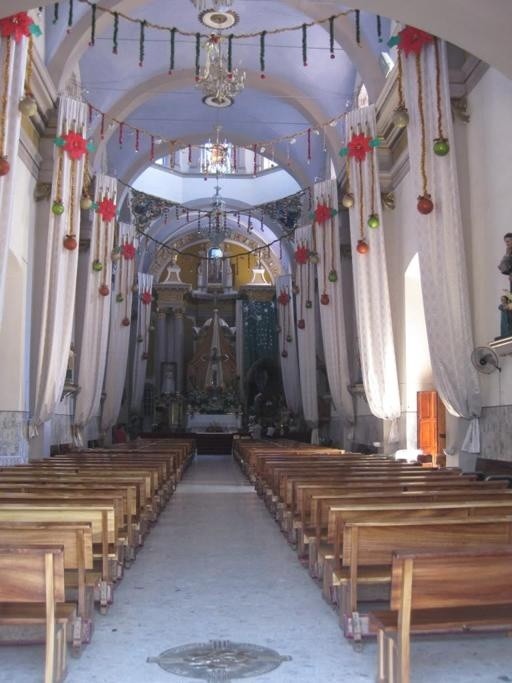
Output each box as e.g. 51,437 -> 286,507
471,347 -> 501,374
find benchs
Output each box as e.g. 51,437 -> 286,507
232,439 -> 512,683
0,437 -> 194,683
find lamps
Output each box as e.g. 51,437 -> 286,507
200,124 -> 232,174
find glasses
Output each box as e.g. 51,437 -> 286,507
200,124 -> 232,174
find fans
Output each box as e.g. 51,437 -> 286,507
471,347 -> 501,374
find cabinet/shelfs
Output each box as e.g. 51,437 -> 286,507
417,391 -> 446,468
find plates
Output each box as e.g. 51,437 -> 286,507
417,391 -> 446,468
